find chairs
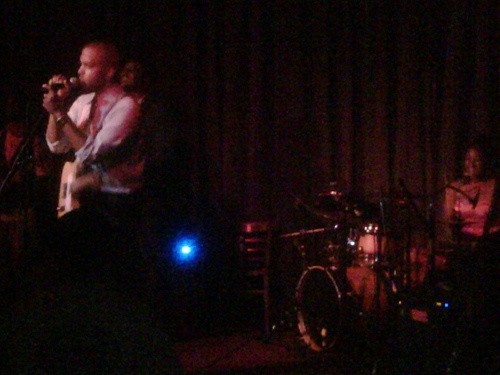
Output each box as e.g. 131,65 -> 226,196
237,221 -> 274,337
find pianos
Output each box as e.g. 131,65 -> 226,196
355,200 -> 482,254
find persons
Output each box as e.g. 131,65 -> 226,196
42,43 -> 144,218
5,107 -> 55,252
443,146 -> 500,245
119,57 -> 167,183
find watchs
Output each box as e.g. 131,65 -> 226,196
56,115 -> 68,128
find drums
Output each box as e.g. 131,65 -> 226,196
357,223 -> 406,269
294,266 -> 350,353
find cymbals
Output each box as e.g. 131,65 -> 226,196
318,188 -> 349,198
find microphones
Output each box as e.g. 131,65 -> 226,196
40,77 -> 78,93
